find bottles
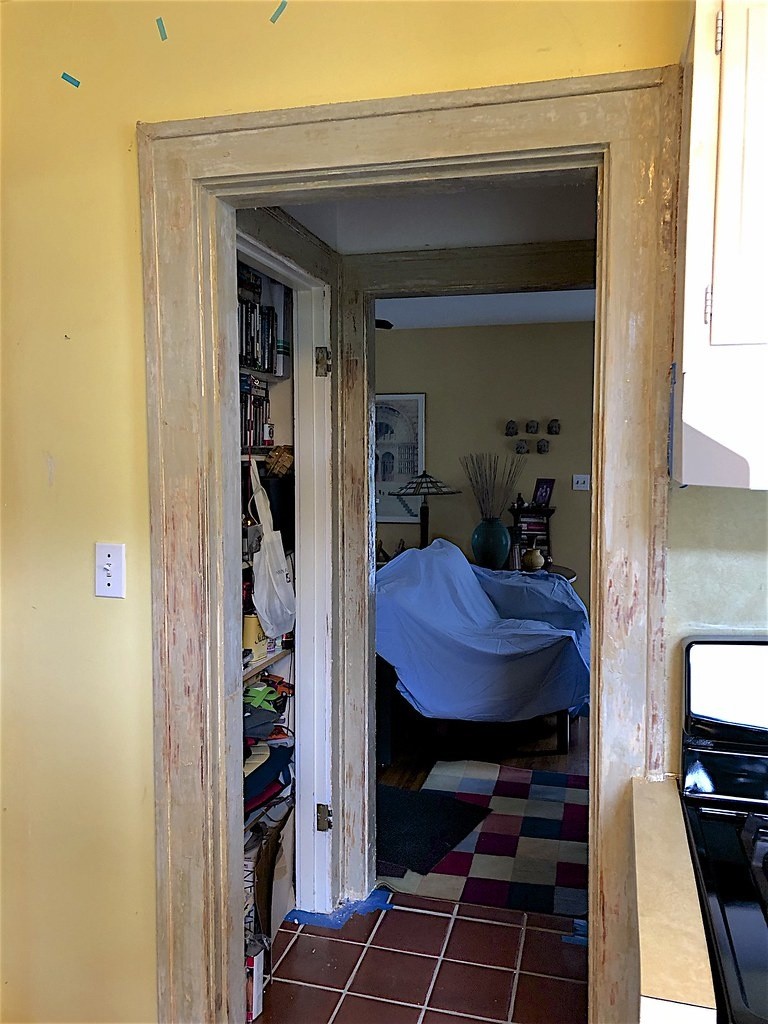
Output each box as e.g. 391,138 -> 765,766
522,548 -> 544,570
516,492 -> 525,508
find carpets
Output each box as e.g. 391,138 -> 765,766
375,760 -> 588,920
375,782 -> 494,876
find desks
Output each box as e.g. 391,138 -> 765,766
486,562 -> 577,589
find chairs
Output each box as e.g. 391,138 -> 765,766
374,539 -> 590,764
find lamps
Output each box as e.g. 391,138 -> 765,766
387,470 -> 464,552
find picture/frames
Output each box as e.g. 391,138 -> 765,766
375,392 -> 427,525
533,477 -> 557,507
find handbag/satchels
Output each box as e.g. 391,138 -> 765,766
250,458 -> 296,637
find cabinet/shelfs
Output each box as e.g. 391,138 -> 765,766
507,506 -> 557,562
673,0 -> 768,491
239,363 -> 295,835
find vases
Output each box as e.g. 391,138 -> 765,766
471,519 -> 511,570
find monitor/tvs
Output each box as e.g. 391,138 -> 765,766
680,635 -> 768,745
